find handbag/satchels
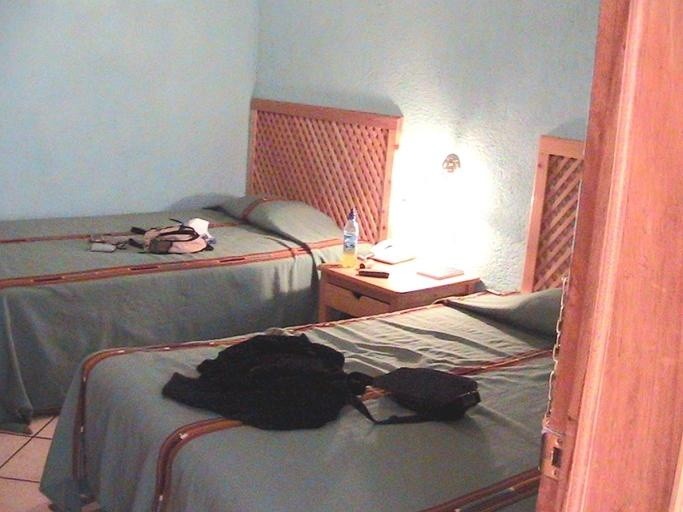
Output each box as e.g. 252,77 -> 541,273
343,365 -> 484,427
125,223 -> 214,255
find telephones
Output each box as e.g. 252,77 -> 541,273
369,239 -> 415,264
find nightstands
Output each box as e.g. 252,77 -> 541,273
317,256 -> 480,323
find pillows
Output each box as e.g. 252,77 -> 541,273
203,194 -> 346,280
432,290 -> 560,337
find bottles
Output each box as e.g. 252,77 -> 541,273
341,209 -> 359,267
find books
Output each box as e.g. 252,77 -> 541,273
356,241 -> 375,261
416,264 -> 464,280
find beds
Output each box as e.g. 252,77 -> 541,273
36,133 -> 586,512
1,96 -> 404,435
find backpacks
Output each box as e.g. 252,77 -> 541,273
160,330 -> 349,431
